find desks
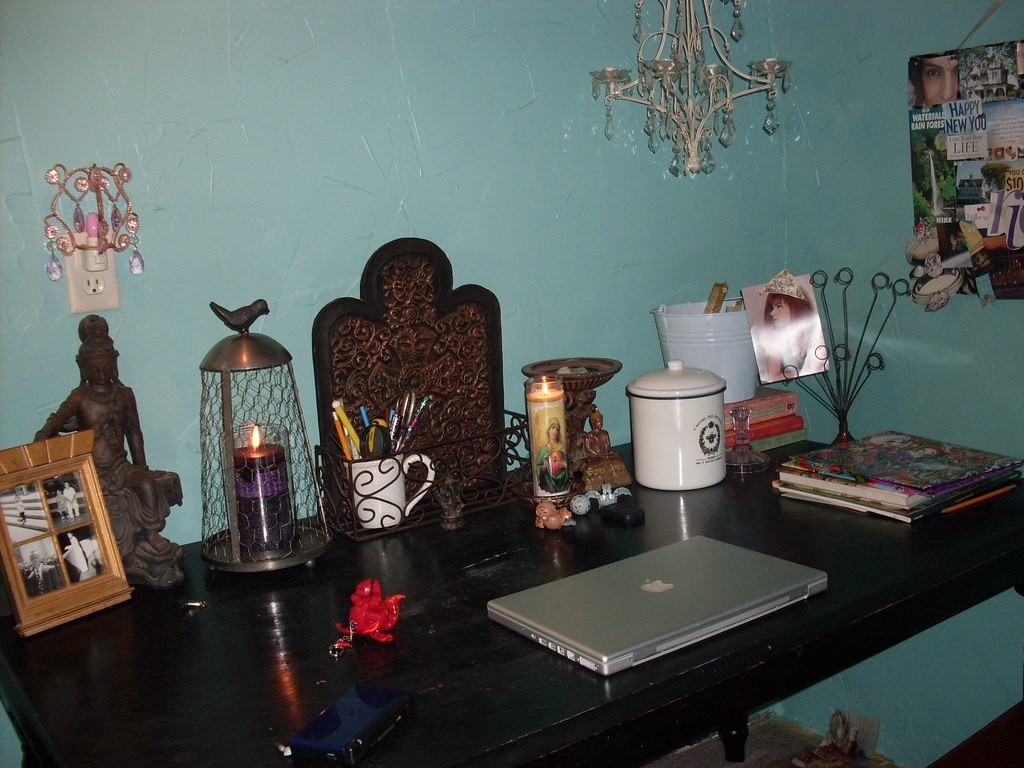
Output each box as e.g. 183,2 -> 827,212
0,440 -> 1024,768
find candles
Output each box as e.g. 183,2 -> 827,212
235,444 -> 292,552
524,376 -> 571,506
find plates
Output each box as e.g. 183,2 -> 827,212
511,479 -> 585,510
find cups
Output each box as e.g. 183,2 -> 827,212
524,376 -> 571,498
344,454 -> 436,531
219,424 -> 299,564
626,360 -> 728,492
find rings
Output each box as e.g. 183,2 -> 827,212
912,267 -> 965,313
904,215 -> 940,266
909,250 -> 944,281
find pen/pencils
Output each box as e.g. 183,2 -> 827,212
325,392 -> 432,461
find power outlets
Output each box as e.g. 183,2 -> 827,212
61,231 -> 120,313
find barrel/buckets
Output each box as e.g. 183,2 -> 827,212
651,300 -> 757,404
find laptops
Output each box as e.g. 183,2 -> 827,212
487,536 -> 829,676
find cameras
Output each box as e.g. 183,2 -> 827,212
288,683 -> 415,768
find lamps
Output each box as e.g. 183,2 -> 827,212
588,0 -> 793,177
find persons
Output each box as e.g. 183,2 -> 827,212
757,283 -> 823,381
32,314 -> 185,589
945,226 -> 968,263
909,54 -> 961,110
582,411 -> 632,493
14,480 -> 102,595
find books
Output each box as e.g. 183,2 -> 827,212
723,385 -> 808,457
771,429 -> 1024,525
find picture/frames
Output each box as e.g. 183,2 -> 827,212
0,429 -> 135,637
